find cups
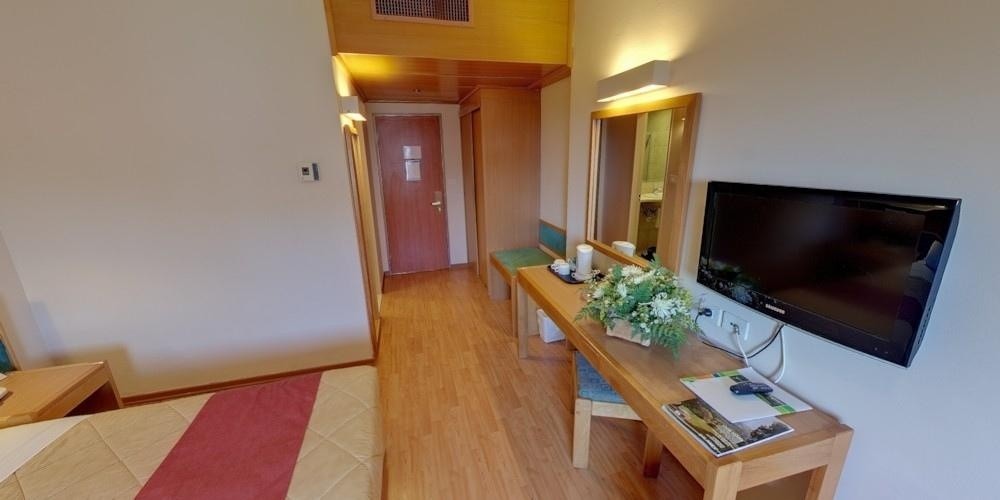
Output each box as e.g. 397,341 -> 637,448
551,259 -> 566,269
555,262 -> 571,276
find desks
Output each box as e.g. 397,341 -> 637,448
516,261 -> 854,500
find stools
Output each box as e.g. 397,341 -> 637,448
564,350 -> 662,479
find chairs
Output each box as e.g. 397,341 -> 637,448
489,220 -> 567,333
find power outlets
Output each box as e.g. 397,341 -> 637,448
695,302 -> 723,327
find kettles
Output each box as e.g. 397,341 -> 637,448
576,244 -> 594,275
611,240 -> 636,257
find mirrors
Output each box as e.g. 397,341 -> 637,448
583,90 -> 704,281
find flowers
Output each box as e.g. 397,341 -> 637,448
573,251 -> 701,363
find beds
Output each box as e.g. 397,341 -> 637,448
1,364 -> 384,500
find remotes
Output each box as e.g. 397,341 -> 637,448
730,382 -> 773,396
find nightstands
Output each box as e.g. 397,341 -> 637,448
0,359 -> 124,425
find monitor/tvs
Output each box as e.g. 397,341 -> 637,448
696,181 -> 962,368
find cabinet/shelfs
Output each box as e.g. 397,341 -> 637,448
457,84 -> 541,287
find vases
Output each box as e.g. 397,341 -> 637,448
606,317 -> 653,348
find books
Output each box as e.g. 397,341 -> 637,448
661,397 -> 794,458
679,366 -> 813,424
